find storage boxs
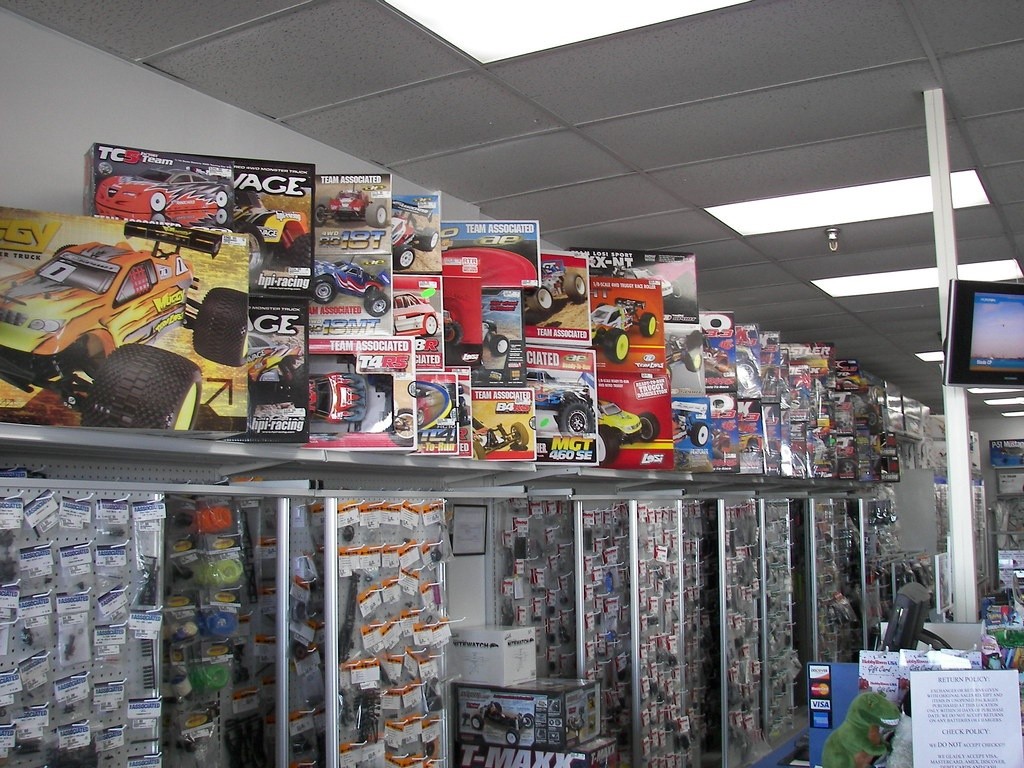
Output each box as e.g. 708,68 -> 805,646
0,143 -> 982,484
449,626 -> 621,768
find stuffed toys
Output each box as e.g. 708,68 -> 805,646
821,691 -> 900,768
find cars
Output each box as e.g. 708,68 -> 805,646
307,372 -> 368,422
391,291 -> 438,338
615,266 -> 675,297
93,167 -> 233,213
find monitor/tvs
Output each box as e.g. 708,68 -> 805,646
941,280 -> 1024,389
882,582 -> 954,652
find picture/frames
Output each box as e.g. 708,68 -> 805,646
451,503 -> 487,557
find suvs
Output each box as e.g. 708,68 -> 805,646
392,200 -> 439,271
0,219 -> 250,429
523,259 -> 587,313
597,398 -> 660,466
307,258 -> 392,317
590,296 -> 659,364
311,186 -> 388,228
224,191 -> 311,277
